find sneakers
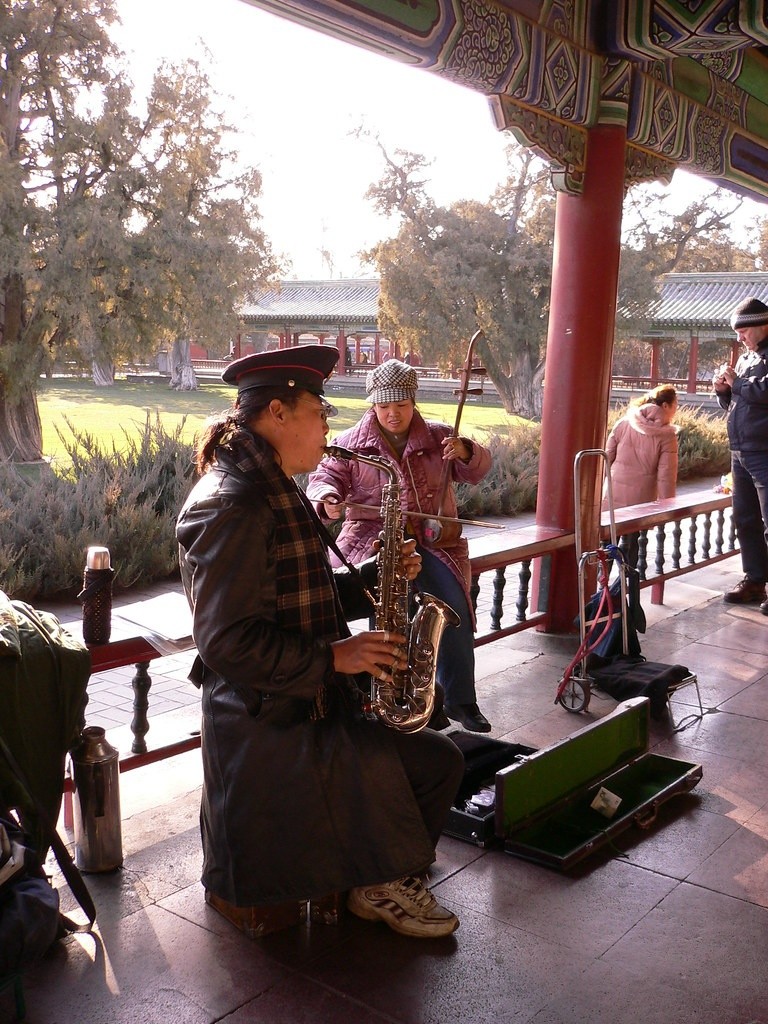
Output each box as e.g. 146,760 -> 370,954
348,876 -> 460,939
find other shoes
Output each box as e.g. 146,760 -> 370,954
758,599 -> 768,613
725,576 -> 767,603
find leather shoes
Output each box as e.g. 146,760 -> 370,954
443,701 -> 491,733
428,709 -> 450,731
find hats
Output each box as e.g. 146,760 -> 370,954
734,299 -> 768,330
221,344 -> 340,395
364,358 -> 418,404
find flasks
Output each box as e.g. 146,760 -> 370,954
83,547 -> 113,641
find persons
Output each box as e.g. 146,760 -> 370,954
176,344 -> 465,938
600,385 -> 680,584
714,297 -> 768,614
306,359 -> 492,733
344,346 -> 421,373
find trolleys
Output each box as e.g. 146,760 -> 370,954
558,448 -> 705,730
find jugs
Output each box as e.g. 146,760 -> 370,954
69,726 -> 124,870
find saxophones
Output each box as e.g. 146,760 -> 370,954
319,444 -> 460,734
